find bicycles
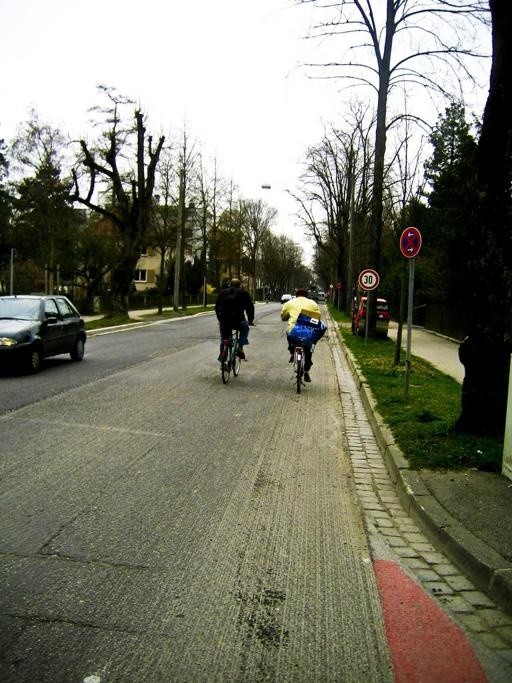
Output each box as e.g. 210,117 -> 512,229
220,323 -> 256,383
289,341 -> 310,394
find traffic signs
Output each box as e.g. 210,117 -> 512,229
358,268 -> 380,291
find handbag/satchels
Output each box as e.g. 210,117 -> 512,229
290,313 -> 327,344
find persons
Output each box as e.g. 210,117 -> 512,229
214,277 -> 254,362
280,288 -> 320,382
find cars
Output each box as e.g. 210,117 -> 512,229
358,296 -> 391,322
0,293 -> 86,374
281,293 -> 293,303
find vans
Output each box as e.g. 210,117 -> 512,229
318,291 -> 325,300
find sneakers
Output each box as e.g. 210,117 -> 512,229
304,372 -> 311,382
220,347 -> 246,371
288,355 -> 295,362
